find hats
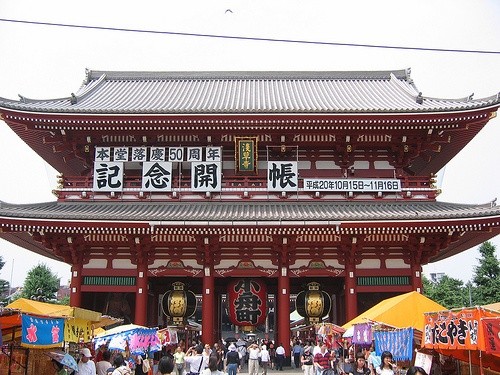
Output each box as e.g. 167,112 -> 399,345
236,339 -> 245,345
296,340 -> 300,344
81,348 -> 92,358
304,345 -> 312,353
191,341 -> 196,344
228,343 -> 237,352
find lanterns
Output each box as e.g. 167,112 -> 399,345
225,279 -> 270,332
295,281 -> 332,325
162,281 -> 198,329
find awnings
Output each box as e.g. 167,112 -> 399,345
315,290 -> 500,375
0,298 -> 180,375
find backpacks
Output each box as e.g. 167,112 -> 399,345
117,368 -> 133,375
142,358 -> 151,373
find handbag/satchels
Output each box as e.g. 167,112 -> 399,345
186,371 -> 200,375
301,365 -> 305,371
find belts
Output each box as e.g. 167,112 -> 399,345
249,359 -> 259,360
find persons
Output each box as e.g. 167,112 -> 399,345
52,335 -> 474,375
